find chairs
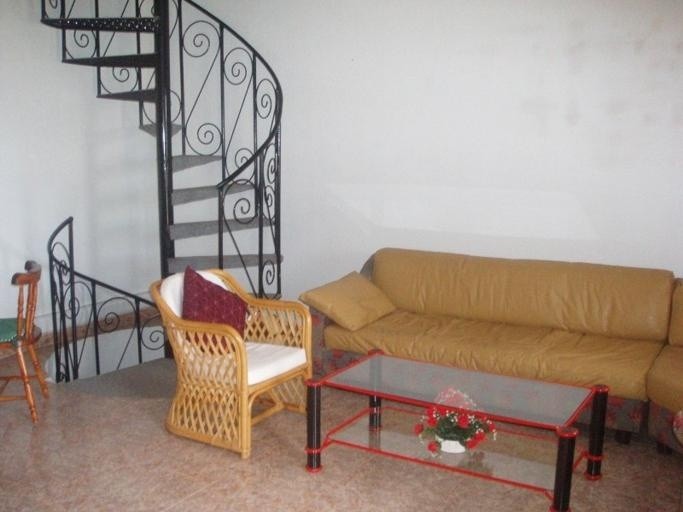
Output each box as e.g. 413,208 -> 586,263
0,259 -> 49,425
148,269 -> 312,459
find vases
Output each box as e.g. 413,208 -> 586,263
435,434 -> 465,454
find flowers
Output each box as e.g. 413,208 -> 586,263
416,388 -> 497,452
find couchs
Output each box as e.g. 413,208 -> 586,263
647,278 -> 682,460
323,248 -> 676,455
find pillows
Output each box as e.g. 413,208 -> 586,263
181,264 -> 248,354
298,271 -> 396,330
